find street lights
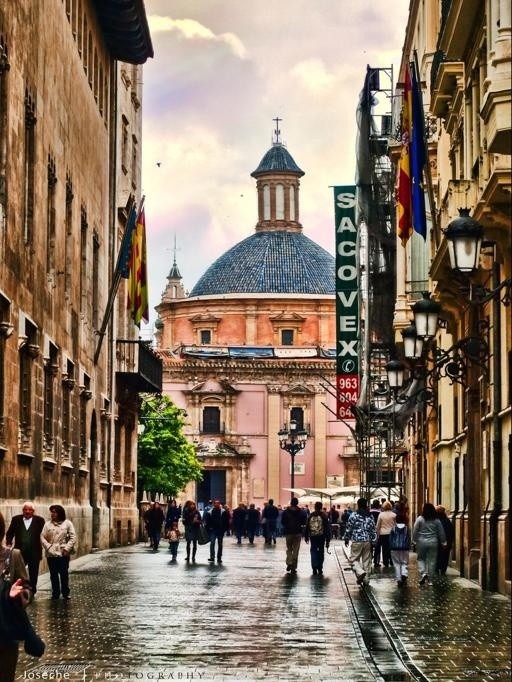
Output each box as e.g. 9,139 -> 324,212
278,418 -> 308,504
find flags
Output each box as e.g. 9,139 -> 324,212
410,69 -> 426,242
118,207 -> 137,276
393,64 -> 414,247
125,208 -> 151,329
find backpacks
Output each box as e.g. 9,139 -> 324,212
310,512 -> 324,537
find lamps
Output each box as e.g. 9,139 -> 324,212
373,207 -> 512,417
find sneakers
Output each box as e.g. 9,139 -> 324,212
419,574 -> 434,585
185,556 -> 222,563
356,573 -> 369,587
286,564 -> 323,575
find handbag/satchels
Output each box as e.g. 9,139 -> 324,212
198,520 -> 211,545
65,534 -> 77,555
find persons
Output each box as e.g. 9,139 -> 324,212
143,498 -> 454,585
40,505 -> 77,601
1,513 -> 32,682
6,502 -> 45,594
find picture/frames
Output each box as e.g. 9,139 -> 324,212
290,463 -> 306,476
